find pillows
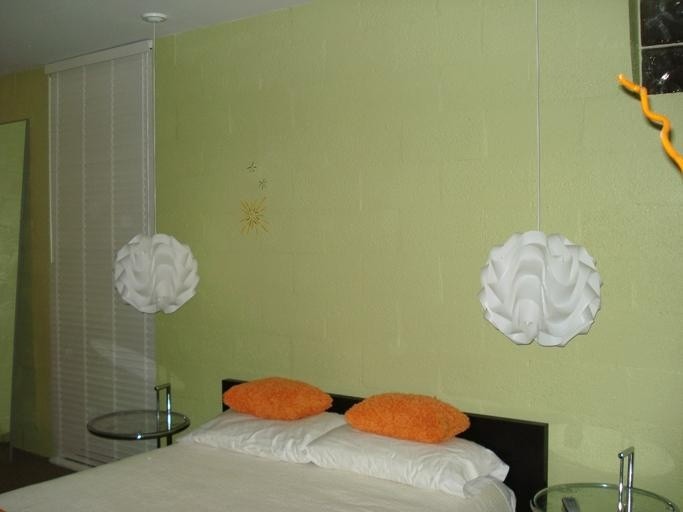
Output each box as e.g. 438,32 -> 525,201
344,391 -> 470,443
221,376 -> 334,421
303,420 -> 510,499
175,407 -> 348,466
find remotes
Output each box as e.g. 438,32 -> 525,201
562,496 -> 580,512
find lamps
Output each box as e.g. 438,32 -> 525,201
478,0 -> 604,348
113,12 -> 200,316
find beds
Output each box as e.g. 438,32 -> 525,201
1,379 -> 548,512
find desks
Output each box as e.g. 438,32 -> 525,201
87,382 -> 190,448
529,447 -> 679,512
